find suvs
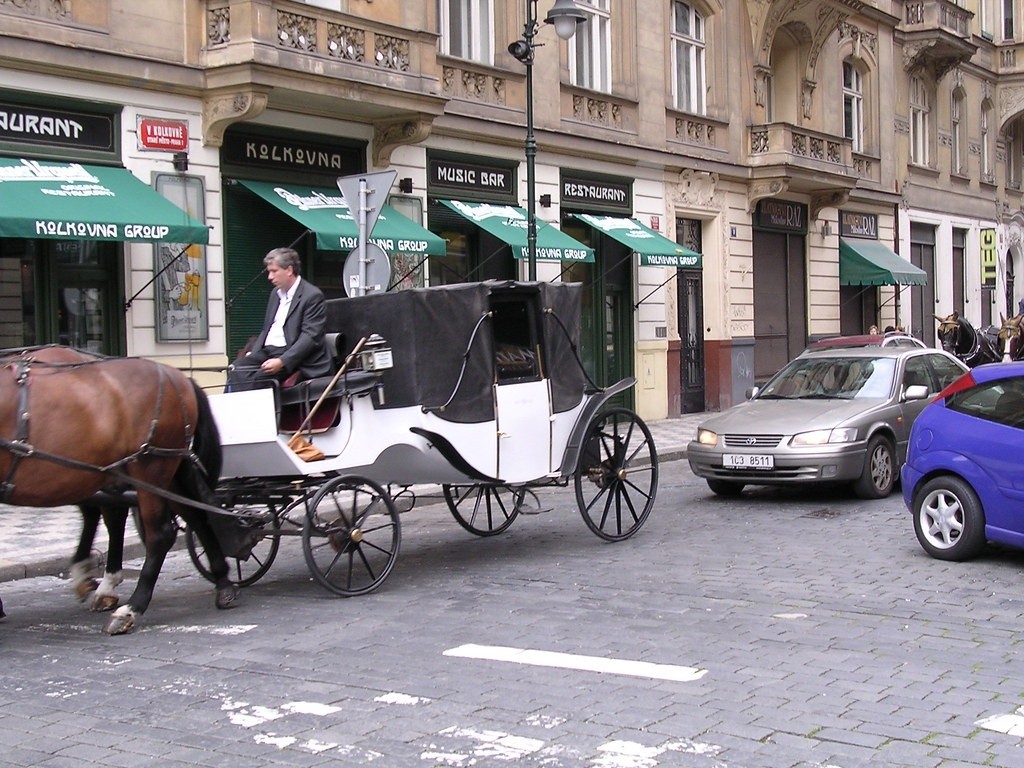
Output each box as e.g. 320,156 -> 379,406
899,361 -> 1024,562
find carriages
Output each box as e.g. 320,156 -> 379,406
0,277 -> 659,636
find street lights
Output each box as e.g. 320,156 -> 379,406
508,1 -> 588,281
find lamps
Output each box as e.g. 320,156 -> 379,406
400,177 -> 412,193
540,194 -> 551,207
173,151 -> 188,171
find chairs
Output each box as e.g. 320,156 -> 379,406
995,391 -> 1024,425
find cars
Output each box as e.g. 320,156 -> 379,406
688,346 -> 971,502
798,332 -> 929,352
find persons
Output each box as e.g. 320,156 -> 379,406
224,248 -> 333,392
864,324 -> 909,347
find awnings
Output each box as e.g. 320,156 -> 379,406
1,159 -> 210,305
226,181 -> 447,310
839,237 -> 928,311
437,201 -> 595,283
571,213 -> 701,307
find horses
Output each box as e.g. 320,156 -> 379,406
0,344 -> 242,635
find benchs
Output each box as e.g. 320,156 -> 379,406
279,370 -> 385,407
